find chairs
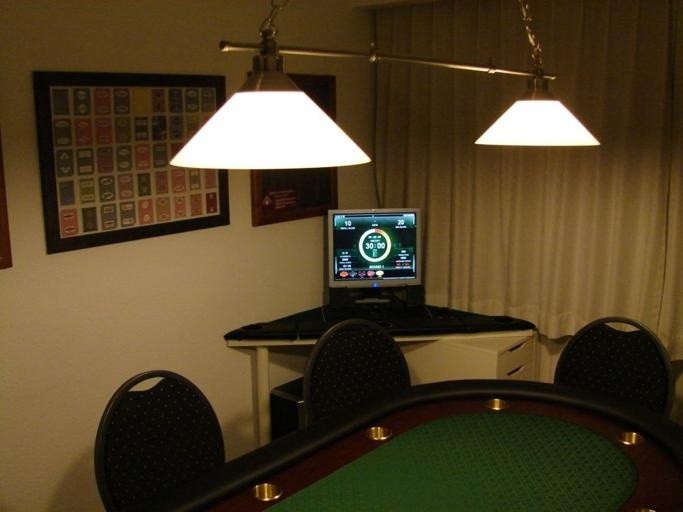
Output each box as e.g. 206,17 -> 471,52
90,366 -> 226,512
305,318 -> 408,440
550,315 -> 676,425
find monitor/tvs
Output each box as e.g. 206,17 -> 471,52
328,208 -> 422,288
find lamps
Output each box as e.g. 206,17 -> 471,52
168,1 -> 602,177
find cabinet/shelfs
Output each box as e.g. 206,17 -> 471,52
406,330 -> 538,387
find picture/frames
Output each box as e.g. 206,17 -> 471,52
32,67 -> 230,258
245,70 -> 338,228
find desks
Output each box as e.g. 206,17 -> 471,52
174,375 -> 681,511
222,296 -> 538,445
